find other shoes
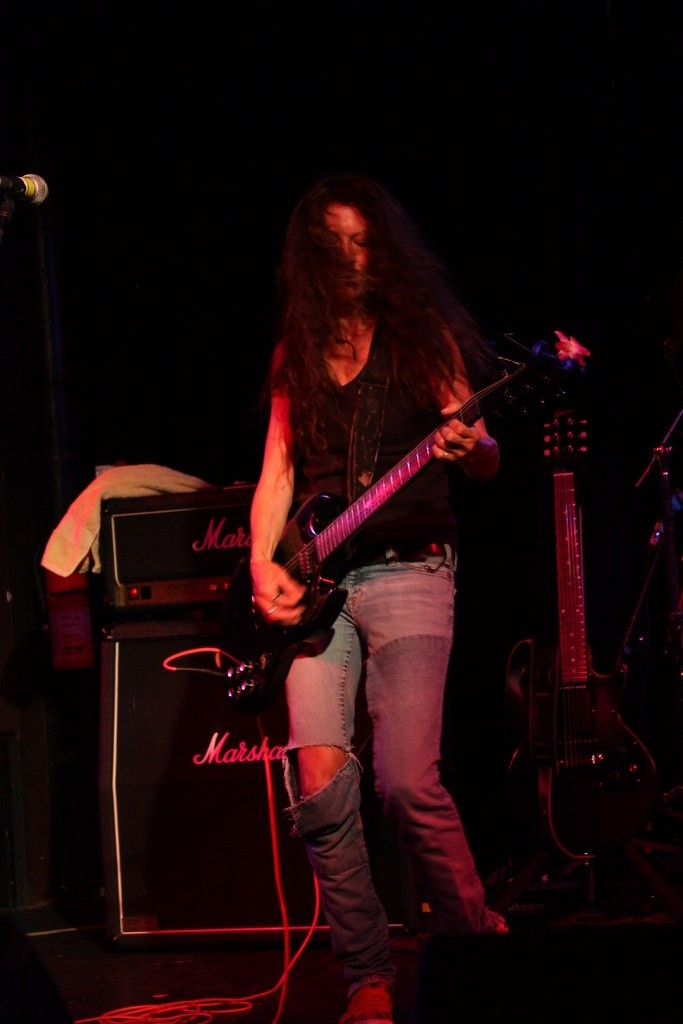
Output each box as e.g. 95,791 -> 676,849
479,907 -> 510,938
337,985 -> 393,1024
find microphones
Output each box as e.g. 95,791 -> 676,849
0,173 -> 48,206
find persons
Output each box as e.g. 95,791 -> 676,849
248,172 -> 511,1024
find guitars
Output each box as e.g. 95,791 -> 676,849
208,332 -> 562,714
535,397 -> 659,859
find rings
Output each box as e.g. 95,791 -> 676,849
264,603 -> 279,618
443,451 -> 449,460
271,591 -> 283,604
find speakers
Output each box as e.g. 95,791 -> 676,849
101,615 -> 421,948
